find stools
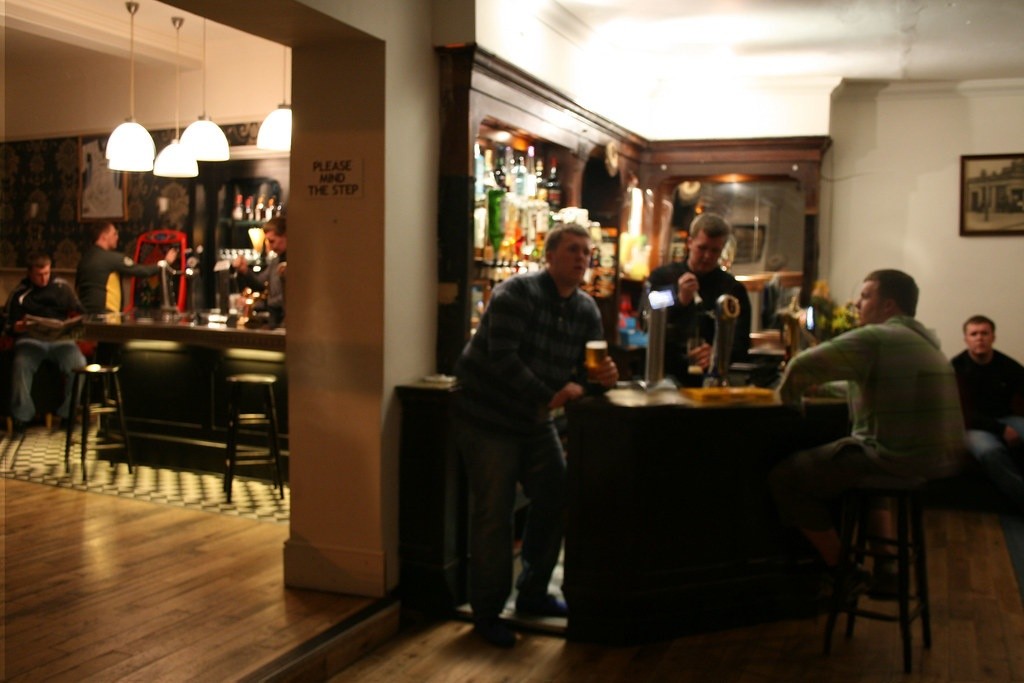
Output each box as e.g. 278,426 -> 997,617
222,374 -> 284,504
826,486 -> 932,671
64,365 -> 132,482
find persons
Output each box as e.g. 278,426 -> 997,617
454,222 -> 620,646
761,266 -> 793,328
0,252 -> 86,432
642,214 -> 752,381
777,270 -> 965,616
77,219 -> 181,402
227,217 -> 286,311
951,316 -> 1024,509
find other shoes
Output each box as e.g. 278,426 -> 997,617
516,592 -> 569,617
472,613 -> 515,648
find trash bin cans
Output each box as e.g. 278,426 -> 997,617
392,372 -> 471,609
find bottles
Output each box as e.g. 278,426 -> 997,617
473,136 -> 598,270
231,194 -> 282,222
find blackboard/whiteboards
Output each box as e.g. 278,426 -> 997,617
130,230 -> 187,313
1,120 -> 262,271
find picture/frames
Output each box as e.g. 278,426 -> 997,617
960,152 -> 1024,237
77,134 -> 129,222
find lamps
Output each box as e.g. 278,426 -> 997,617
180,17 -> 230,162
105,2 -> 155,172
154,17 -> 199,177
254,45 -> 293,152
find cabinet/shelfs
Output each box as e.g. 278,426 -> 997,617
212,177 -> 280,304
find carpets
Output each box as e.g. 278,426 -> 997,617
998,502 -> 1024,600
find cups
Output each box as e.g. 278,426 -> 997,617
585,340 -> 608,383
